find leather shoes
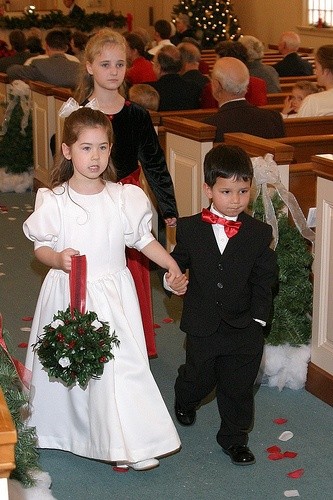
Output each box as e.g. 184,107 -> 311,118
221,444 -> 256,466
174,395 -> 196,427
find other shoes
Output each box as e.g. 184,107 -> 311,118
117,457 -> 159,471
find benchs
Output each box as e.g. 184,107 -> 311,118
158,49 -> 333,226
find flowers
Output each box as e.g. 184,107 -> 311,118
31,304 -> 120,387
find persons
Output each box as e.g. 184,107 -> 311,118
159,145 -> 279,466
0,0 -> 333,117
50,29 -> 178,362
23,108 -> 187,471
199,57 -> 285,142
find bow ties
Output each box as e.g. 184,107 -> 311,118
201,208 -> 242,239
